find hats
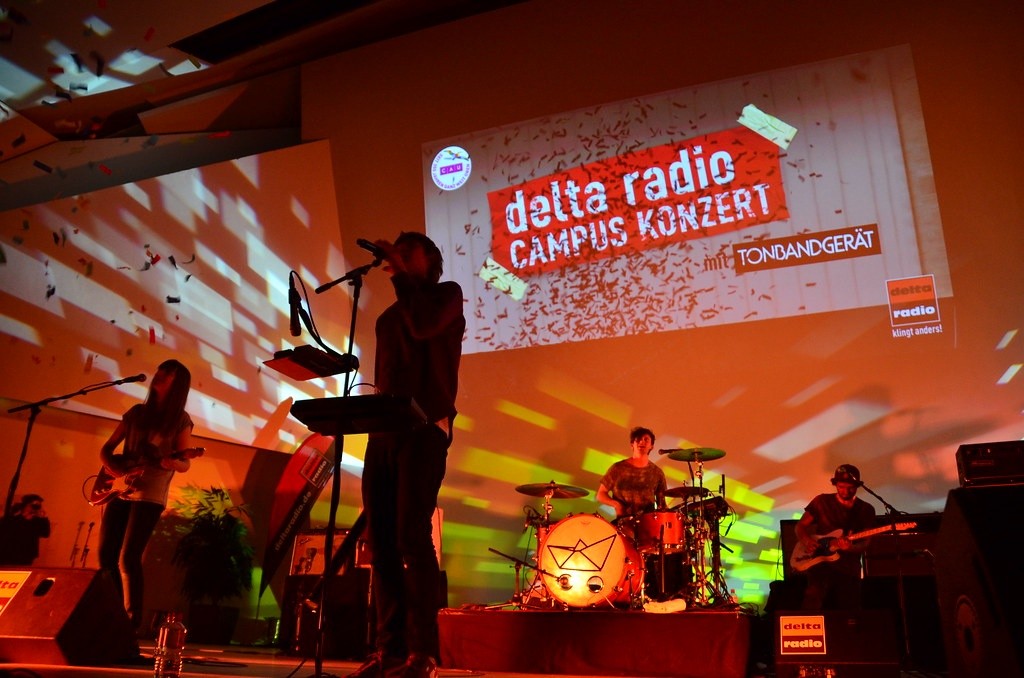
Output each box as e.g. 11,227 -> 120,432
830,464 -> 864,487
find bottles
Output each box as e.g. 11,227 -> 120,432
153,612 -> 186,677
729,588 -> 738,603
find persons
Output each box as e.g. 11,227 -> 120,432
97,359 -> 194,628
344,231 -> 467,678
794,464 -> 875,610
595,427 -> 667,610
0,494 -> 50,566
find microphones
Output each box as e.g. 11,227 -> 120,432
559,577 -> 568,584
837,466 -> 859,486
658,449 -> 683,454
289,275 -> 301,337
358,239 -> 385,258
522,509 -> 532,534
305,548 -> 317,571
115,374 -> 146,385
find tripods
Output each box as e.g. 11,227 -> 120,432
670,453 -> 736,610
481,548 -> 560,612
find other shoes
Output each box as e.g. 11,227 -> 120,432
348,652 -> 407,678
383,661 -> 430,678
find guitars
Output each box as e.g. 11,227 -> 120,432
790,521 -> 917,575
91,446 -> 207,506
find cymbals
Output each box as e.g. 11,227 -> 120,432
664,487 -> 710,498
667,447 -> 726,461
514,483 -> 590,499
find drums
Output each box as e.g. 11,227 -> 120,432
539,519 -> 560,548
537,513 -> 646,609
610,516 -> 637,540
636,510 -> 688,555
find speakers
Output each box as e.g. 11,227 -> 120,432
356,508 -> 446,568
282,568 -> 375,660
290,529 -> 362,574
933,483 -> 1024,678
0,564 -> 141,669
773,607 -> 902,678
